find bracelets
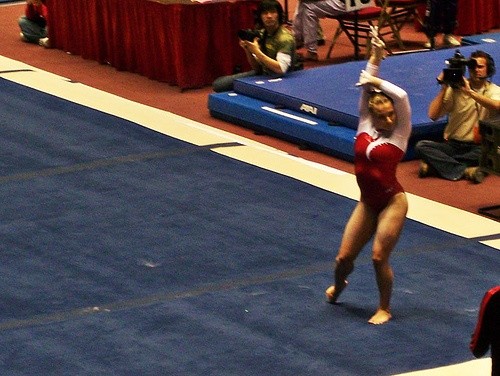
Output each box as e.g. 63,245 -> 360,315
469,90 -> 479,99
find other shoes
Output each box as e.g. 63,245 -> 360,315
418,162 -> 428,178
306,49 -> 318,61
316,39 -> 325,46
469,166 -> 487,182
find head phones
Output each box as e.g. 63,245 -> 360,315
487,55 -> 496,77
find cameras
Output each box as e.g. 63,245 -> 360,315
237,30 -> 260,42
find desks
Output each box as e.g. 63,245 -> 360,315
43,0 -> 267,87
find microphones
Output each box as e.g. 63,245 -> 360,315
479,76 -> 488,79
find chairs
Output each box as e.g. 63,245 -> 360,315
374,0 -> 418,44
327,0 -> 389,59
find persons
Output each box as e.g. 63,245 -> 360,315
469,286 -> 500,376
416,49 -> 500,182
323,24 -> 415,325
18,0 -> 51,48
291,0 -> 462,61
212,0 -> 303,93
